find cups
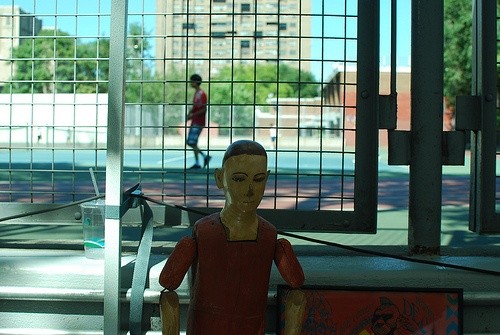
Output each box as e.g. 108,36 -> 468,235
80,200 -> 105,259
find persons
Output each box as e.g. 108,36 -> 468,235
186,74 -> 211,170
159,140 -> 306,335
270,123 -> 278,149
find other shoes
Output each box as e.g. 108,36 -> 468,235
203,156 -> 211,168
190,164 -> 201,169
